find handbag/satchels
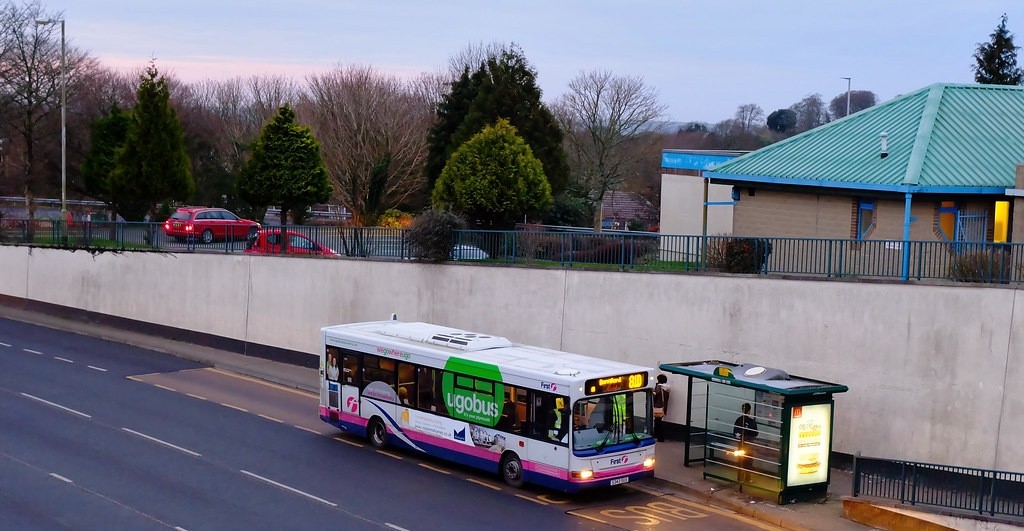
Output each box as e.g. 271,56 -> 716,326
654,407 -> 665,418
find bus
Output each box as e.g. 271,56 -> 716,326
319,313 -> 656,495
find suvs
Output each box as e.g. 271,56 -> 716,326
164,207 -> 261,244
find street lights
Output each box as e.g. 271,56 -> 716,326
841,76 -> 851,116
35,18 -> 68,249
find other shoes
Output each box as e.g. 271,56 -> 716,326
657,438 -> 663,442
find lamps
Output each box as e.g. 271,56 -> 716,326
732,186 -> 740,201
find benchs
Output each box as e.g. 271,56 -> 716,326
708,441 -> 778,462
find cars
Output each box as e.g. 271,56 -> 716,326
245,229 -> 342,254
449,243 -> 492,259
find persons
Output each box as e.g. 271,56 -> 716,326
326,354 -> 339,381
613,394 -> 626,434
653,374 -> 669,442
734,403 -> 758,482
496,392 -> 517,433
547,402 -> 568,438
